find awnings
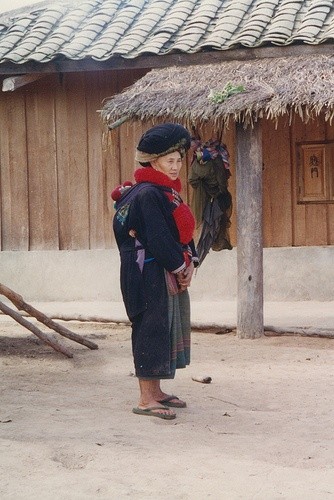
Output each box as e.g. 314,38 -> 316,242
96,55 -> 334,130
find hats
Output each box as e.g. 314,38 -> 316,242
135,123 -> 192,167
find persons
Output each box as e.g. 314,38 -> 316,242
111,124 -> 201,421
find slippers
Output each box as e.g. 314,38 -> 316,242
155,395 -> 186,407
132,406 -> 176,419
